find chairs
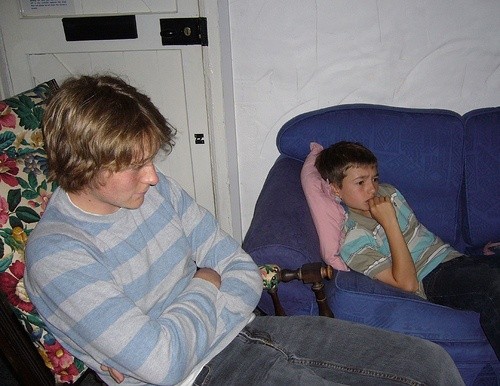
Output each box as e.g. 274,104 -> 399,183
0,79 -> 334,386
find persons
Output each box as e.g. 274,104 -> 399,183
313,141 -> 499,365
23,73 -> 467,386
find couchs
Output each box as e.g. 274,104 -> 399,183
238,103 -> 499,386
